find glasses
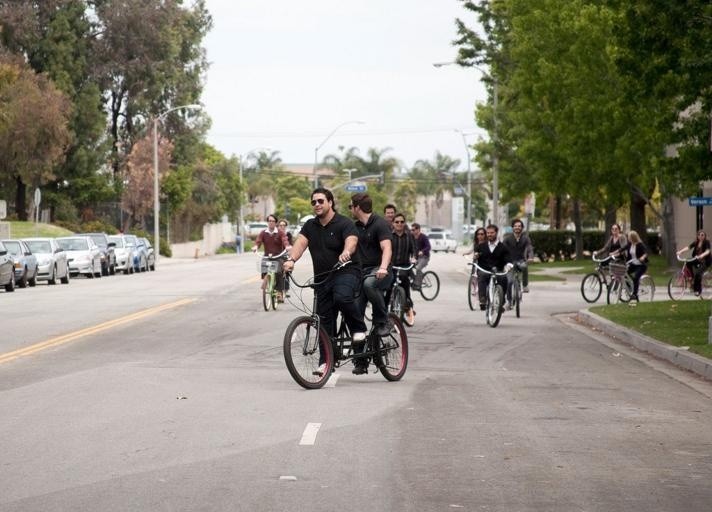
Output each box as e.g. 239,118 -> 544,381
311,198 -> 325,207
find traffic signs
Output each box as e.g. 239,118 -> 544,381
686,196 -> 712,207
344,185 -> 368,192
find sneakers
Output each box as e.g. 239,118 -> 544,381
351,332 -> 366,342
353,363 -> 367,373
377,319 -> 394,338
313,363 -> 334,374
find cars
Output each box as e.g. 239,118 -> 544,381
75,231 -> 157,274
55,236 -> 102,278
243,217 -> 299,239
0,240 -> 16,292
1,238 -> 39,287
406,220 -> 457,255
20,236 -> 70,284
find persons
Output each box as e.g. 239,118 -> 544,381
253,188 -> 431,374
236,234 -> 242,254
610,231 -> 650,300
593,224 -> 627,290
676,230 -> 712,296
470,218 -> 533,309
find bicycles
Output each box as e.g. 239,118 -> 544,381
507,259 -> 533,319
248,246 -> 293,311
468,257 -> 510,325
668,251 -> 712,300
361,251 -> 442,326
461,252 -> 484,312
276,259 -> 409,384
606,253 -> 656,306
581,250 -> 633,301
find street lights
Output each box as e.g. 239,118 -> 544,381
310,117 -> 370,205
452,126 -> 472,236
151,103 -> 204,265
237,146 -> 274,256
340,168 -> 355,203
430,58 -> 500,232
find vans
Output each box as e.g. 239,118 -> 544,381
463,224 -> 480,235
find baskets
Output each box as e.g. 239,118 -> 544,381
609,263 -> 627,277
258,260 -> 279,274
